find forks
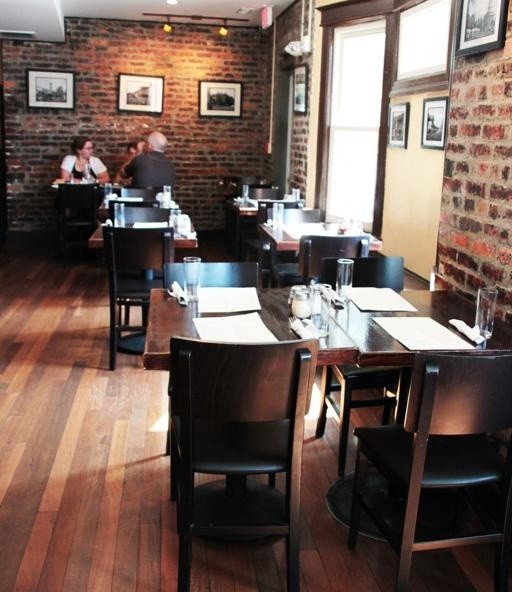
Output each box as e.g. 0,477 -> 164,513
288,315 -> 298,324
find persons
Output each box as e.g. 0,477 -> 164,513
119,131 -> 175,197
115,143 -> 138,186
59,137 -> 111,217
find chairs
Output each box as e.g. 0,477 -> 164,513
103,224 -> 175,371
53,163 -> 420,286
350,348 -> 512,588
167,335 -> 319,590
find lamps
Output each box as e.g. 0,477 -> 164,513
159,11 -> 230,37
283,35 -> 312,58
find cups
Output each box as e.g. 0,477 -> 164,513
293,189 -> 300,202
83,163 -> 91,181
312,284 -> 330,336
475,287 -> 496,339
272,204 -> 285,229
114,202 -> 126,230
169,209 -> 182,237
104,182 -> 113,204
336,258 -> 354,302
183,255 -> 202,304
163,186 -> 172,205
64,172 -> 73,183
243,185 -> 249,198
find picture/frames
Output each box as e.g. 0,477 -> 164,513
197,80 -> 244,119
115,70 -> 165,115
23,65 -> 79,112
290,62 -> 311,117
387,99 -> 409,152
452,0 -> 510,59
419,95 -> 452,151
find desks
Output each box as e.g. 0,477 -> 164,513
302,281 -> 512,477
142,285 -> 360,429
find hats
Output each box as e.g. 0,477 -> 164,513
148,131 -> 168,153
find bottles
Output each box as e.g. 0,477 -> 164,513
292,292 -> 311,318
309,286 -> 320,316
178,215 -> 191,235
287,285 -> 308,313
